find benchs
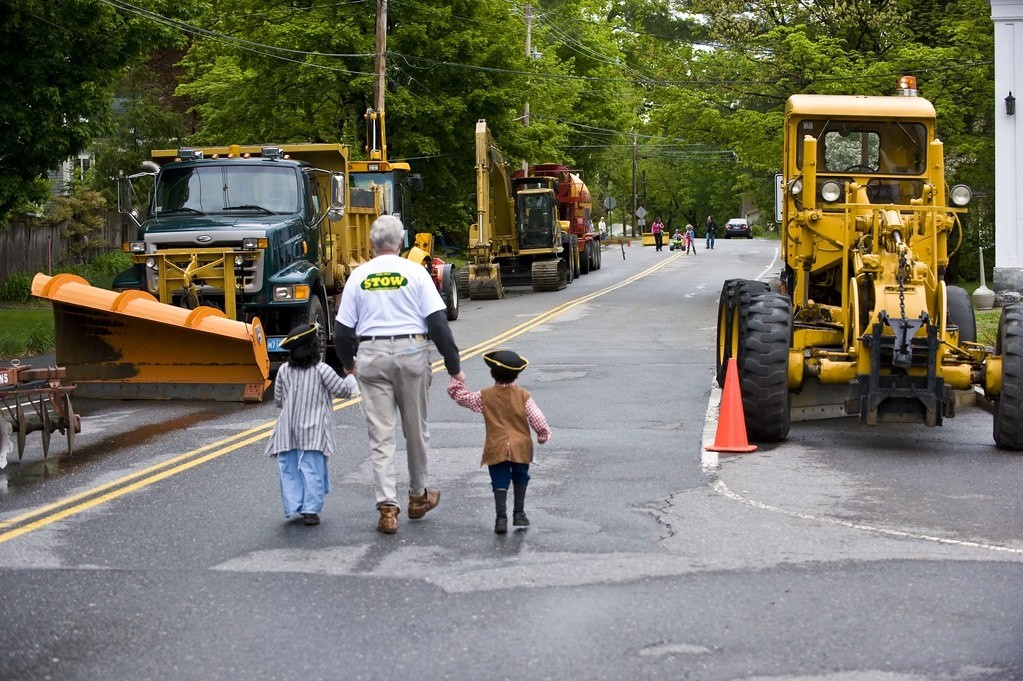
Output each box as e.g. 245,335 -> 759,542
816,183 -> 904,204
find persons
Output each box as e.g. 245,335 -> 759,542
685,225 -> 694,252
332,214 -> 466,533
264,325 -> 360,519
652,217 -> 664,251
673,229 -> 683,241
448,350 -> 551,533
705,216 -> 715,249
598,217 -> 610,246
590,220 -> 595,232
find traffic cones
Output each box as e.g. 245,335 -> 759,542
705,358 -> 758,453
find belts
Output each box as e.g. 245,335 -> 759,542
359,334 -> 428,343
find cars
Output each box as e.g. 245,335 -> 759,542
723,218 -> 753,239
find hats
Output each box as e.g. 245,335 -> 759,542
278,323 -> 317,350
483,350 -> 530,374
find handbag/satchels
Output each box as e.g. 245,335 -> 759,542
660,230 -> 665,236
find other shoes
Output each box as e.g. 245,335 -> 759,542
513,512 -> 530,526
303,513 -> 320,526
287,513 -> 304,523
495,518 -> 507,534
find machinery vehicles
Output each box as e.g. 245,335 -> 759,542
716,75 -> 1023,450
30,100 -> 459,404
457,118 -> 603,300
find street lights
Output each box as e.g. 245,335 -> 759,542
522,45 -> 542,186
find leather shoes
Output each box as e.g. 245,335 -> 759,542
408,487 -> 440,519
377,505 -> 400,534
